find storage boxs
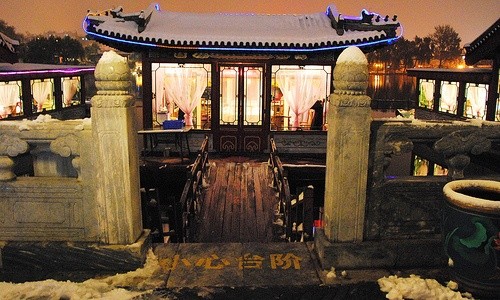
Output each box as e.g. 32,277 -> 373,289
162,119 -> 185,129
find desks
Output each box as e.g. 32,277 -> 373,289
137,125 -> 192,163
